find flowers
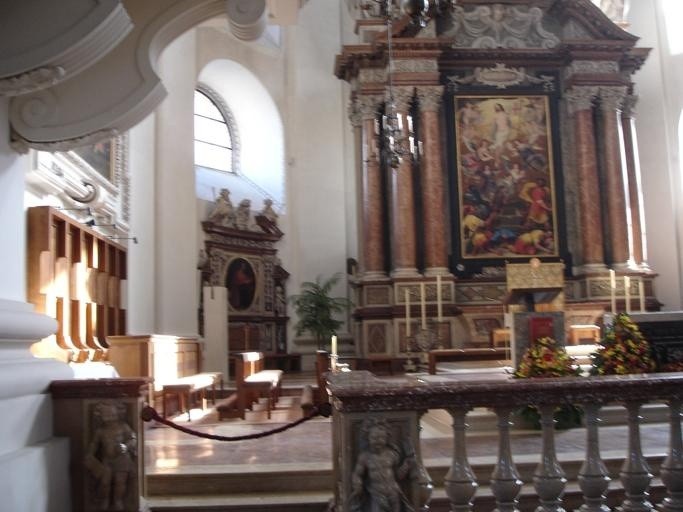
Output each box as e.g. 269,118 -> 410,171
589,314 -> 657,375
504,337 -> 584,379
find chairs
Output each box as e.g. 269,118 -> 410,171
315,349 -> 334,405
235,352 -> 284,420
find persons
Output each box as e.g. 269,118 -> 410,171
204,188 -> 278,235
488,102 -> 522,149
475,164 -> 503,215
463,204 -> 504,257
496,160 -> 529,200
517,103 -> 547,150
348,415 -> 420,512
82,399 -> 139,511
499,229 -> 554,255
518,178 -> 553,232
456,101 -> 483,159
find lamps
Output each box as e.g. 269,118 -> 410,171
364,0 -> 424,169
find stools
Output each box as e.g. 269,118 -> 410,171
570,325 -> 601,345
488,328 -> 511,348
162,372 -> 224,423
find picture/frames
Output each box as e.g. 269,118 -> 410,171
445,90 -> 569,275
